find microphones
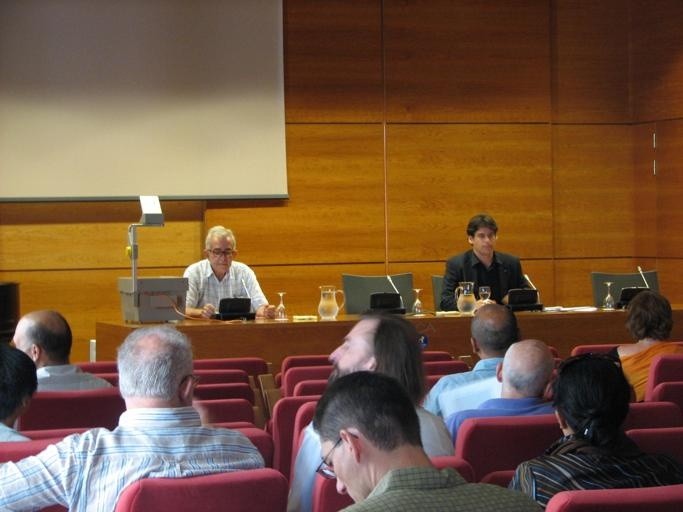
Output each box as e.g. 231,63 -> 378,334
387,274 -> 404,308
637,265 -> 649,288
241,278 -> 256,313
523,274 -> 541,304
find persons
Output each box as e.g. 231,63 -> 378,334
1,309 -> 266,512
439,211 -> 523,312
184,225 -> 278,321
286,291 -> 682,512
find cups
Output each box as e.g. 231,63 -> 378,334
455,282 -> 475,313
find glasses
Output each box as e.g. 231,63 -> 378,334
180,374 -> 200,388
207,248 -> 233,257
315,433 -> 358,480
558,351 -> 622,374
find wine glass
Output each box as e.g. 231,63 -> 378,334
479,286 -> 491,306
603,281 -> 615,311
275,290 -> 286,320
411,288 -> 424,313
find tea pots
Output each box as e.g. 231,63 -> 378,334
318,286 -> 345,320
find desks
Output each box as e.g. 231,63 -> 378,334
89,307 -> 683,377
